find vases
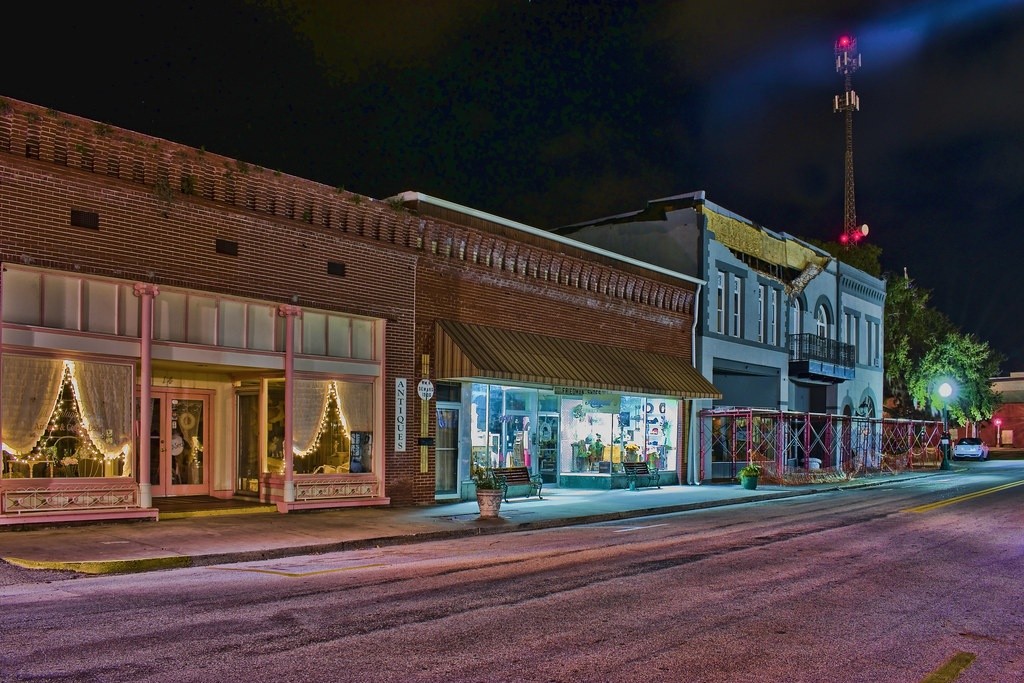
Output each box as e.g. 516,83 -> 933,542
66,464 -> 79,477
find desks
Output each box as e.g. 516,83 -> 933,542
6,459 -> 54,479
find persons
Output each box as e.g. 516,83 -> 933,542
523,422 -> 531,467
332,441 -> 339,457
949,425 -> 958,451
362,436 -> 370,472
46,446 -> 63,468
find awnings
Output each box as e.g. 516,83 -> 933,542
437,322 -> 724,401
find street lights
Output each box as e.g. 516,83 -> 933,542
938,383 -> 952,470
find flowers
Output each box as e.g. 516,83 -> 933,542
61,456 -> 78,466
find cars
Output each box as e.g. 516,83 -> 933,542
954,437 -> 991,461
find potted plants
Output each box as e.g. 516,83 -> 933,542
589,439 -> 603,461
473,468 -> 503,519
575,450 -> 589,470
738,464 -> 761,489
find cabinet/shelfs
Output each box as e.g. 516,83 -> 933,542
646,412 -> 666,469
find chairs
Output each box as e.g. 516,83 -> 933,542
315,462 -> 349,474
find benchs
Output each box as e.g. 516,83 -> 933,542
621,462 -> 660,490
490,466 -> 543,503
